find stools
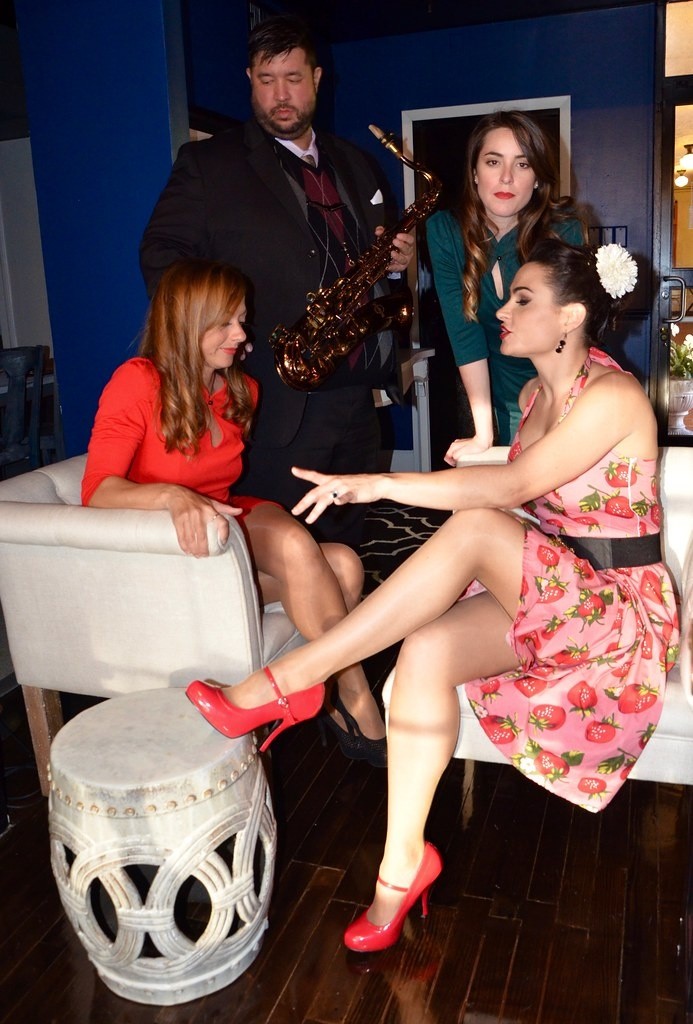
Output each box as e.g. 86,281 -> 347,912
47,686 -> 278,1007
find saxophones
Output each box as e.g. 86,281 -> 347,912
266,121 -> 446,394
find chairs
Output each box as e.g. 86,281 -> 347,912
0,345 -> 48,481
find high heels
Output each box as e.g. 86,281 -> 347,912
185,666 -> 325,756
329,679 -> 388,769
316,705 -> 368,761
343,841 -> 445,953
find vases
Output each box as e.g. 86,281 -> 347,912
667,380 -> 693,436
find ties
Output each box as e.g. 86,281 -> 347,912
300,154 -> 317,168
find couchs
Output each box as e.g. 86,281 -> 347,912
383,447 -> 693,825
0,451 -> 310,795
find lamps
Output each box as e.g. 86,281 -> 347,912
674,144 -> 693,187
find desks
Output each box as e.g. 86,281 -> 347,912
373,348 -> 435,473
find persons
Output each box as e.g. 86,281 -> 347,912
426,111 -> 591,468
82,262 -> 387,768
137,22 -> 415,558
185,195 -> 681,953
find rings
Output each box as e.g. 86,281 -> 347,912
212,513 -> 220,521
329,491 -> 337,499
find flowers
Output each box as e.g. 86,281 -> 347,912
670,324 -> 693,379
595,243 -> 638,300
